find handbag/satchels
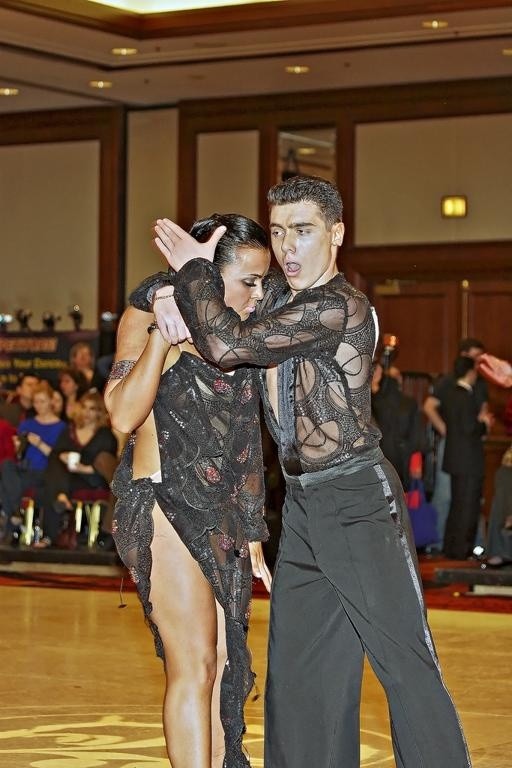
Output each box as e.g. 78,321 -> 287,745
401,476 -> 441,547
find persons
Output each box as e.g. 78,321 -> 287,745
103,214 -> 274,768
1,375 -> 40,458
0,415 -> 20,546
371,333 -> 419,495
485,443 -> 512,566
15,385 -> 69,487
58,369 -> 86,424
128,176 -> 474,767
442,355 -> 497,562
424,339 -> 487,564
32,391 -> 118,550
49,392 -> 65,420
70,343 -> 107,401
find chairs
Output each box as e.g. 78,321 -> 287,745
22,491 -> 45,545
71,491 -> 109,551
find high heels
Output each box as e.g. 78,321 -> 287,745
480,556 -> 507,570
